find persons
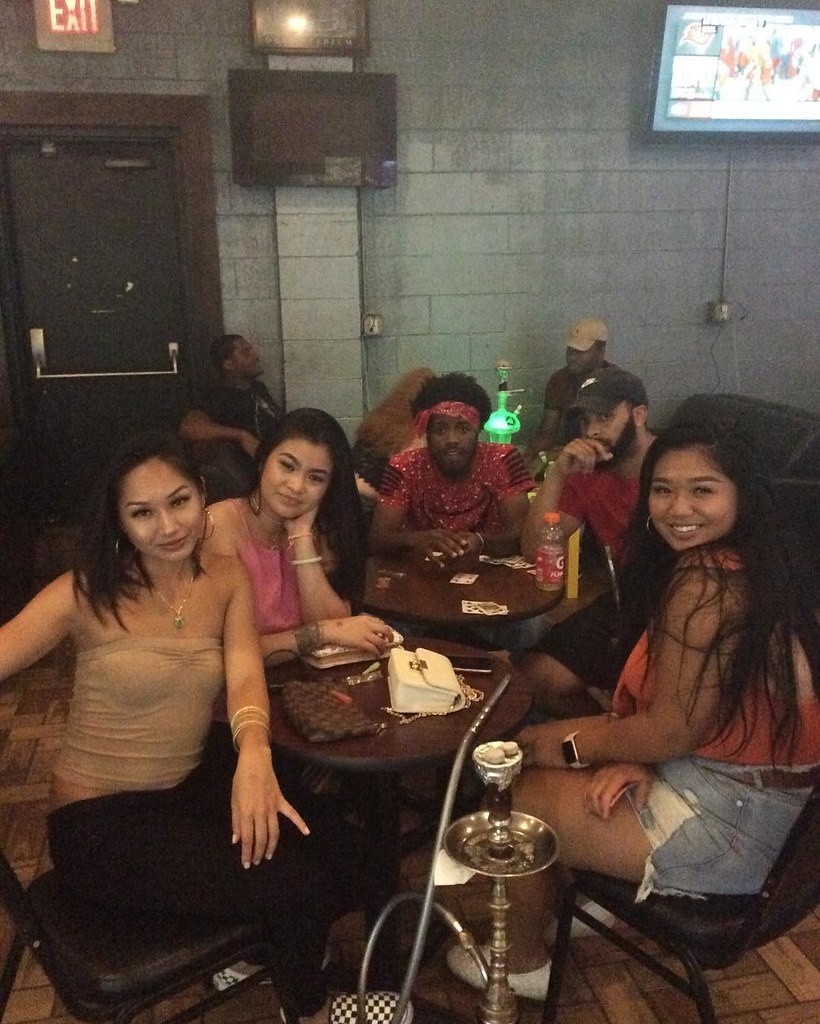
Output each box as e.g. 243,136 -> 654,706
179,334 -> 286,485
373,370 -> 536,568
205,407 -> 394,782
446,421 -> 820,1000
350,368 -> 436,529
0,431 -> 415,1024
520,367 -> 679,714
522,318 -> 620,478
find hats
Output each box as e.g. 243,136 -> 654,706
565,319 -> 608,351
564,367 -> 648,420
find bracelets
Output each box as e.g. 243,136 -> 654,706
294,620 -> 319,655
539,451 -> 547,466
231,706 -> 272,753
475,533 -> 484,554
288,533 -> 312,539
291,557 -> 321,564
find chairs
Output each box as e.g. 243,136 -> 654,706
0,850 -> 304,1024
542,781 -> 820,1024
193,442 -> 258,497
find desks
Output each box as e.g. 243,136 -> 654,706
263,636 -> 529,1024
367,556 -> 565,656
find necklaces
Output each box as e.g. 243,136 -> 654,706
252,491 -> 282,524
154,573 -> 194,628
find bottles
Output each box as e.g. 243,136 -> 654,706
534,513 -> 565,593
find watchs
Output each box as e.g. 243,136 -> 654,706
562,731 -> 592,768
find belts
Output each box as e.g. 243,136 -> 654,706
710,763 -> 820,789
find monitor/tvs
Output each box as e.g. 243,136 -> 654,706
643,0 -> 820,145
225,69 -> 400,190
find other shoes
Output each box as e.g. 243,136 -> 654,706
279,990 -> 414,1024
212,943 -> 329,991
543,900 -> 614,943
446,943 -> 553,999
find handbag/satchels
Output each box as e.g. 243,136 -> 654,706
282,680 -> 382,744
388,647 -> 465,714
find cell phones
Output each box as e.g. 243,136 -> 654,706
447,654 -> 491,673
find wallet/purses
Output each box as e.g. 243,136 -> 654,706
301,614 -> 407,669
